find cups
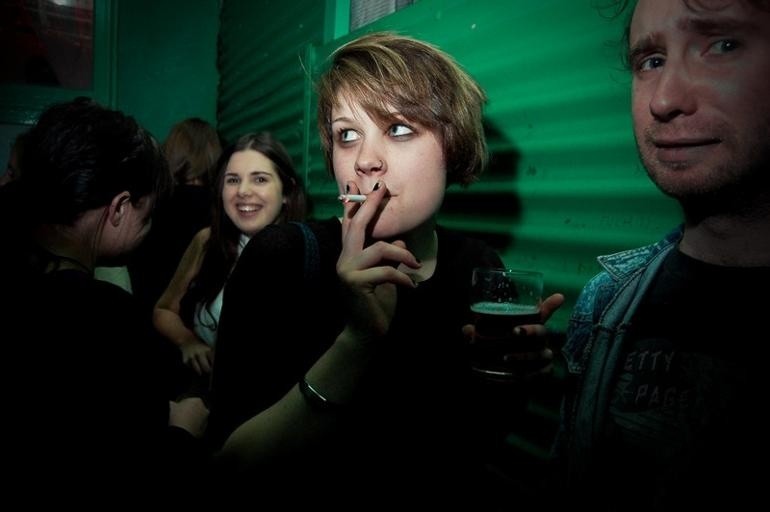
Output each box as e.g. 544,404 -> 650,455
471,269 -> 547,378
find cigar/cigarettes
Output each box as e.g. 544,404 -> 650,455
339,191 -> 367,205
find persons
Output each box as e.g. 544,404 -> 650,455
151,129 -> 314,400
204,30 -> 565,512
128,117 -> 232,306
0,95 -> 224,512
482,0 -> 770,509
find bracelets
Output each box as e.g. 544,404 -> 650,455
298,370 -> 338,415
